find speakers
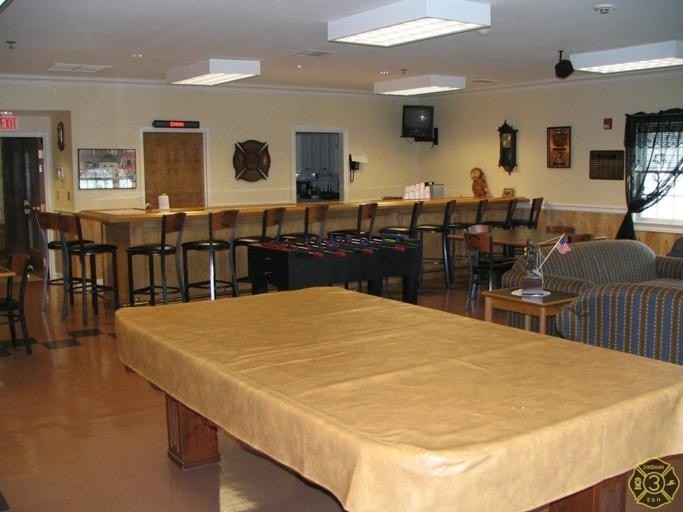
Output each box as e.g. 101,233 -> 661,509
555,59 -> 574,77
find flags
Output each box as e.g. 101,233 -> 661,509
555,239 -> 571,255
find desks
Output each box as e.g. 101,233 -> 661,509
478,288 -> 578,338
112,281 -> 683,512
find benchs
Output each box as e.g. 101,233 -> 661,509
553,279 -> 682,366
502,235 -> 682,339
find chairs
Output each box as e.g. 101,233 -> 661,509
459,232 -> 519,309
0,196 -> 606,359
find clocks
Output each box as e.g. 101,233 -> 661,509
495,118 -> 520,175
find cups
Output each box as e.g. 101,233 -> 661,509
402,183 -> 430,200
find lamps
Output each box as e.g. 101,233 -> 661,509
162,56 -> 262,88
324,0 -> 492,48
570,38 -> 682,75
371,73 -> 466,101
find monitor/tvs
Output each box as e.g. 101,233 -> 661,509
402,104 -> 434,137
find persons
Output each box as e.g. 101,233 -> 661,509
470,168 -> 488,199
